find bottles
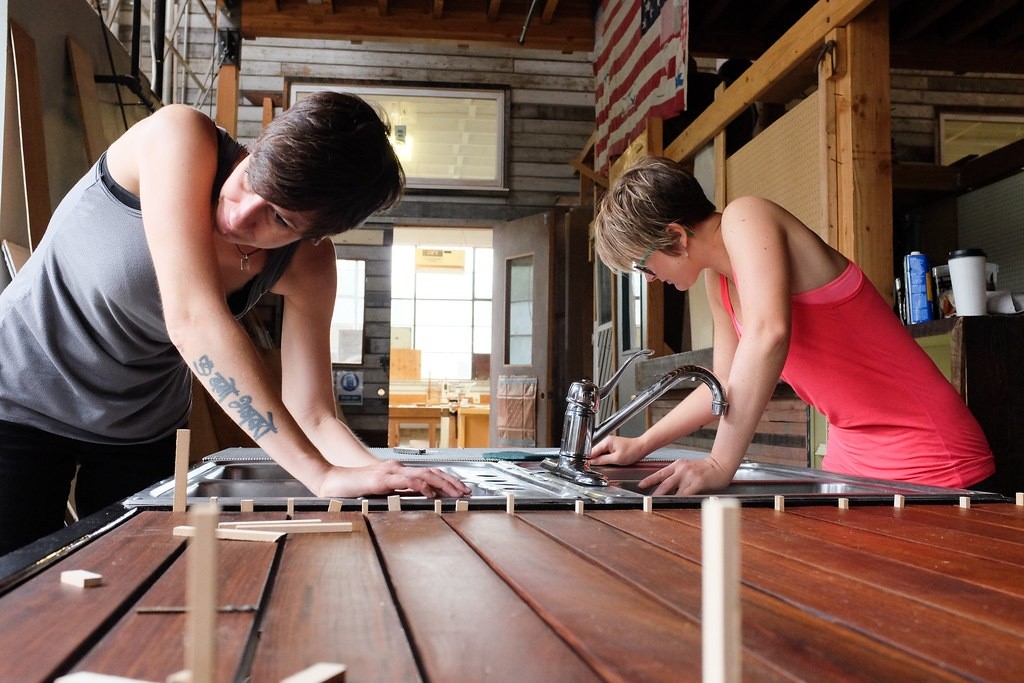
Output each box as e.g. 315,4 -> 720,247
903,251 -> 934,325
932,265 -> 955,320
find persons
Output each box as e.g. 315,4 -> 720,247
0,91 -> 473,553
584,153 -> 1002,497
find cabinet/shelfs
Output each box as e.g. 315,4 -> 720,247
388,392 -> 490,448
806,315 -> 1024,498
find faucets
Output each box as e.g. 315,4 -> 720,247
539,349 -> 730,488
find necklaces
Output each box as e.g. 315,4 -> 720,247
235,243 -> 264,270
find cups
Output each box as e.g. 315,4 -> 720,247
946,247 -> 988,316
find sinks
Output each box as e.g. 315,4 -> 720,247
610,479 -> 1006,504
158,480 -> 317,497
582,461 -> 847,479
205,462 -> 298,482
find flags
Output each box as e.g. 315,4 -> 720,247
569,0 -> 692,177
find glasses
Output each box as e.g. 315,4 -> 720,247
634,223 -> 696,275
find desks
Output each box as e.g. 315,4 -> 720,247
2,444 -> 1023,682
